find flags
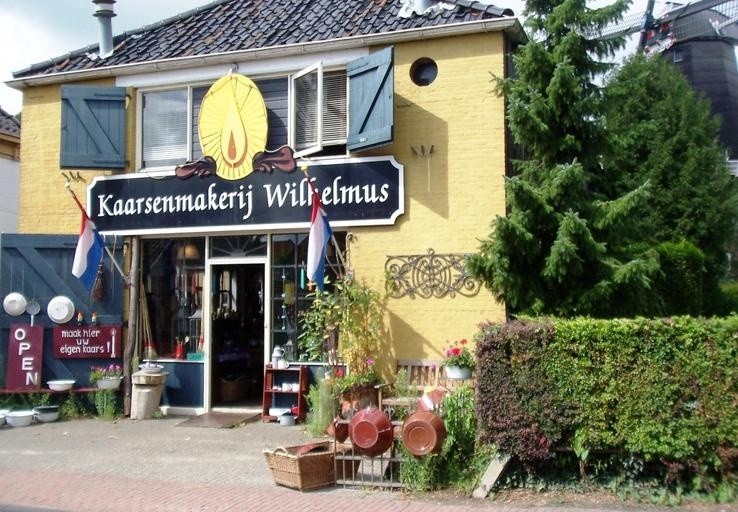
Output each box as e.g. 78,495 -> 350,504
71,212 -> 105,291
306,192 -> 332,294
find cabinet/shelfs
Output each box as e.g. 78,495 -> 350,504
263,363 -> 308,420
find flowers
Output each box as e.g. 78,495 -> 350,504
443,339 -> 477,368
330,358 -> 381,393
90,363 -> 122,379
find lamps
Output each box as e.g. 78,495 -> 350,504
177,239 -> 200,261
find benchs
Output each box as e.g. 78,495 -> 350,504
374,359 -> 462,416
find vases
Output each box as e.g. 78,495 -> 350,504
446,363 -> 472,378
0,404 -> 60,427
97,377 -> 123,390
340,381 -> 444,459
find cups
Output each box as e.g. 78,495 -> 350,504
291,384 -> 299,392
272,346 -> 290,371
281,383 -> 291,392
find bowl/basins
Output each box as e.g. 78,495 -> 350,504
47,295 -> 76,325
6,410 -> 35,428
400,411 -> 448,457
33,405 -> 60,422
3,291 -> 28,317
348,408 -> 394,458
444,364 -> 474,379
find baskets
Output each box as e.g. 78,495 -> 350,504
264,440 -> 363,492
